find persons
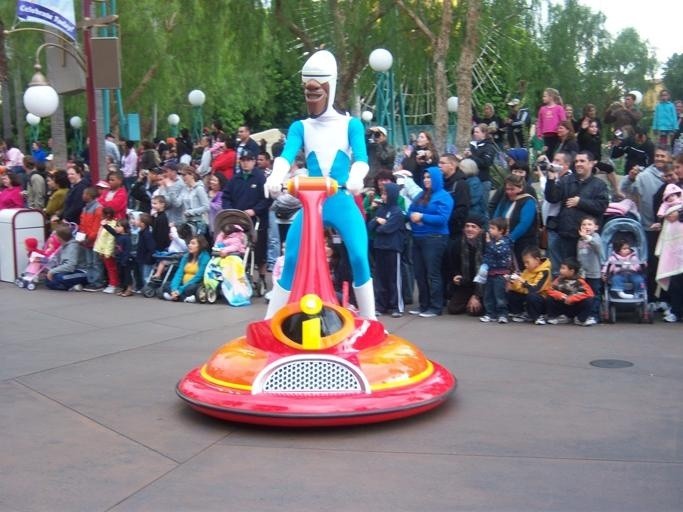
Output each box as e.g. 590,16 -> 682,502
562,103 -> 603,138
99,125 -> 292,306
604,93 -> 641,147
0,134 -> 111,291
470,96 -> 531,147
536,87 -> 566,154
653,90 -> 681,148
261,48 -> 377,323
359,122 -> 681,327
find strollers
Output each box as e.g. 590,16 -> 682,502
598,207 -> 654,325
197,208 -> 264,304
141,221 -> 203,299
17,223 -> 80,290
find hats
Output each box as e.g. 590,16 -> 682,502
507,99 -> 519,105
368,125 -> 387,136
506,148 -> 526,163
178,166 -> 194,175
239,150 -> 255,161
459,159 -> 477,174
159,162 -> 175,171
463,215 -> 485,227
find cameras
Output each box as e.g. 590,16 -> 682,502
638,166 -> 645,171
144,169 -> 149,176
612,101 -> 621,105
532,161 -> 546,171
615,131 -> 625,141
366,129 -> 381,138
550,164 -> 563,172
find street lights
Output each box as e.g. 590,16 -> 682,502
66,114 -> 84,157
24,112 -> 39,154
22,41 -> 99,189
368,47 -> 393,132
167,113 -> 180,138
188,90 -> 207,142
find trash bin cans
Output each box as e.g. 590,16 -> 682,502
0,207 -> 48,283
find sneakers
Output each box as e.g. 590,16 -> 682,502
103,285 -> 133,296
409,307 -> 438,317
657,302 -> 683,322
184,295 -> 196,303
72,282 -> 104,292
479,313 -> 598,326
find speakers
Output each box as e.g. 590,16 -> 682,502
89,37 -> 121,89
